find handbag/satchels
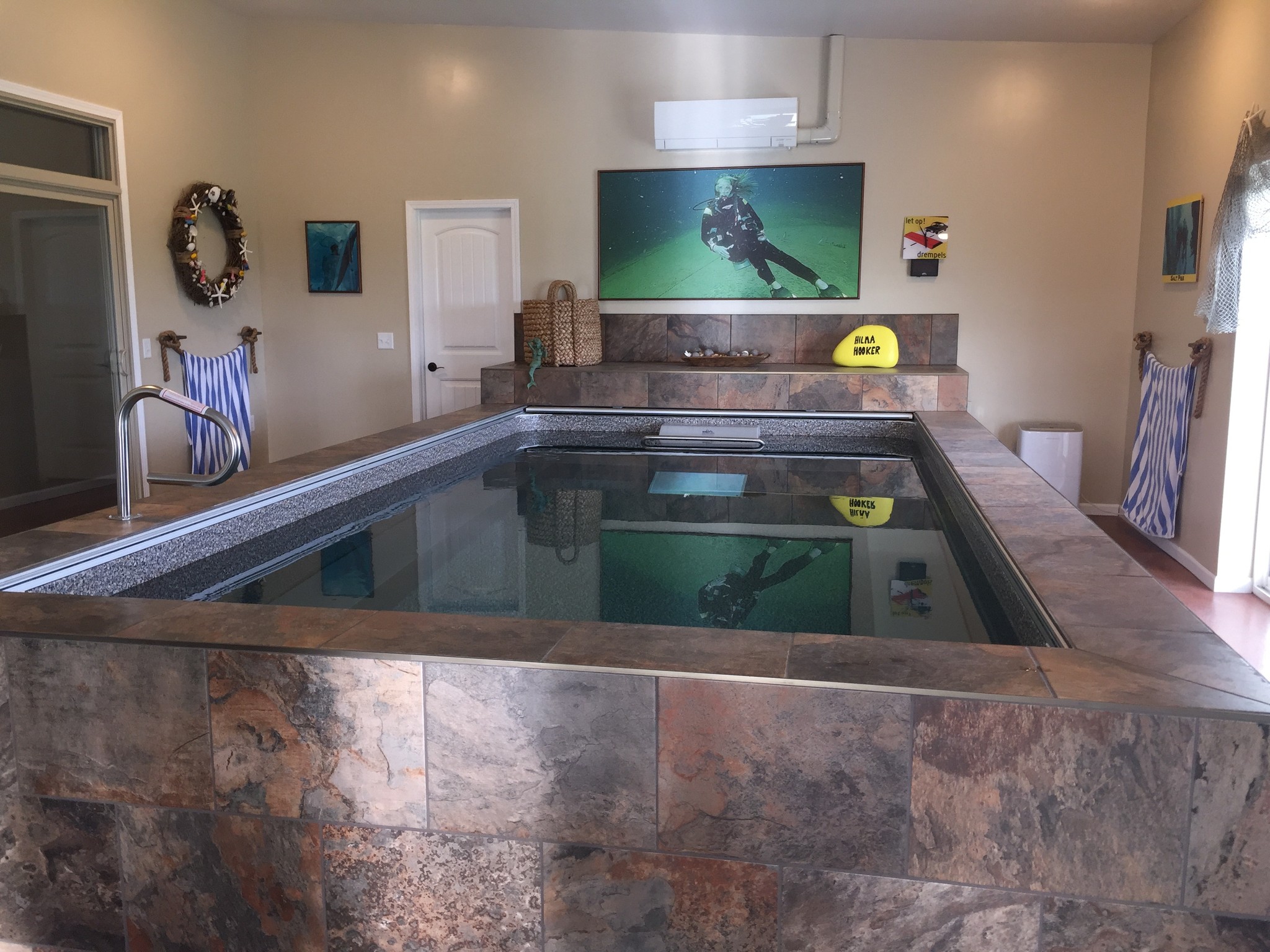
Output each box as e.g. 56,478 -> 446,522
527,489 -> 603,565
522,280 -> 602,367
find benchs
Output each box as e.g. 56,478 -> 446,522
481,315 -> 969,413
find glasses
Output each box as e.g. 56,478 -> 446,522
715,183 -> 733,193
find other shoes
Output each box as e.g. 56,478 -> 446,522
819,284 -> 849,298
772,285 -> 797,298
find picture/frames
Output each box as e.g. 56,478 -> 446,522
1161,195 -> 1204,283
305,220 -> 362,294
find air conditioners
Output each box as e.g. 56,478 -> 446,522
654,97 -> 799,151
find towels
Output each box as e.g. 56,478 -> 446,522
1119,351 -> 1198,538
177,344 -> 254,477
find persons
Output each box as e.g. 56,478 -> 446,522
700,175 -> 845,298
699,537 -> 837,633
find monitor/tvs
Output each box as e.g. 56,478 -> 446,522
596,528 -> 853,635
597,161 -> 864,300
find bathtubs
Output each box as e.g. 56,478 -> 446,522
4,409 -> 1269,951
